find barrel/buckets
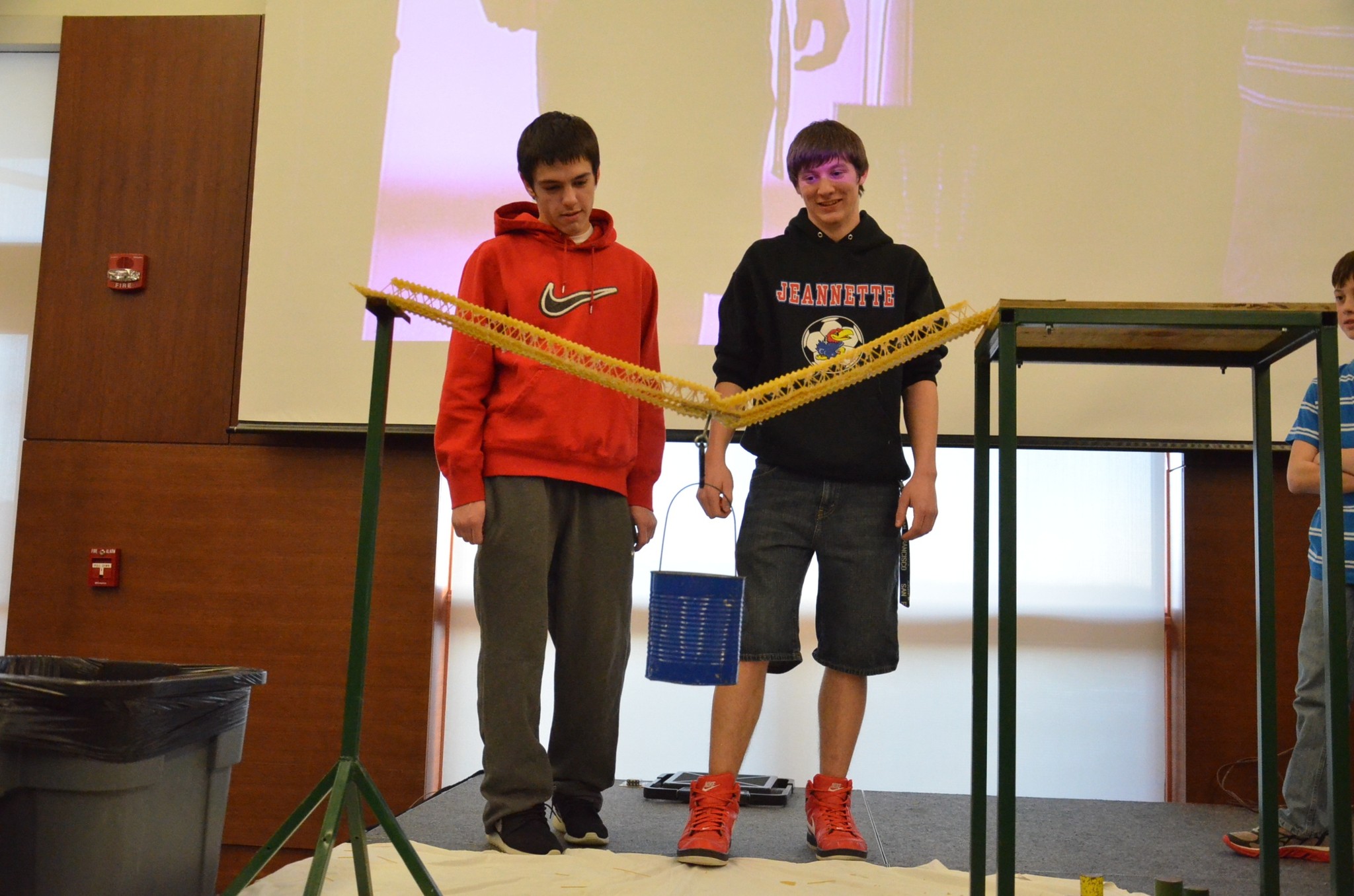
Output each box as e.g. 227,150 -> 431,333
645,571 -> 747,686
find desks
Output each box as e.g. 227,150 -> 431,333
970,300 -> 1354,895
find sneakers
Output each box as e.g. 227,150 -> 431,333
485,801 -> 563,855
677,772 -> 741,865
805,773 -> 868,861
1223,824 -> 1331,862
550,796 -> 609,845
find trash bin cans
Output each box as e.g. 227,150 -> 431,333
0,653 -> 268,896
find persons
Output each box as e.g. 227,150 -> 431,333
433,112 -> 666,857
1224,252 -> 1354,860
677,122 -> 949,866
